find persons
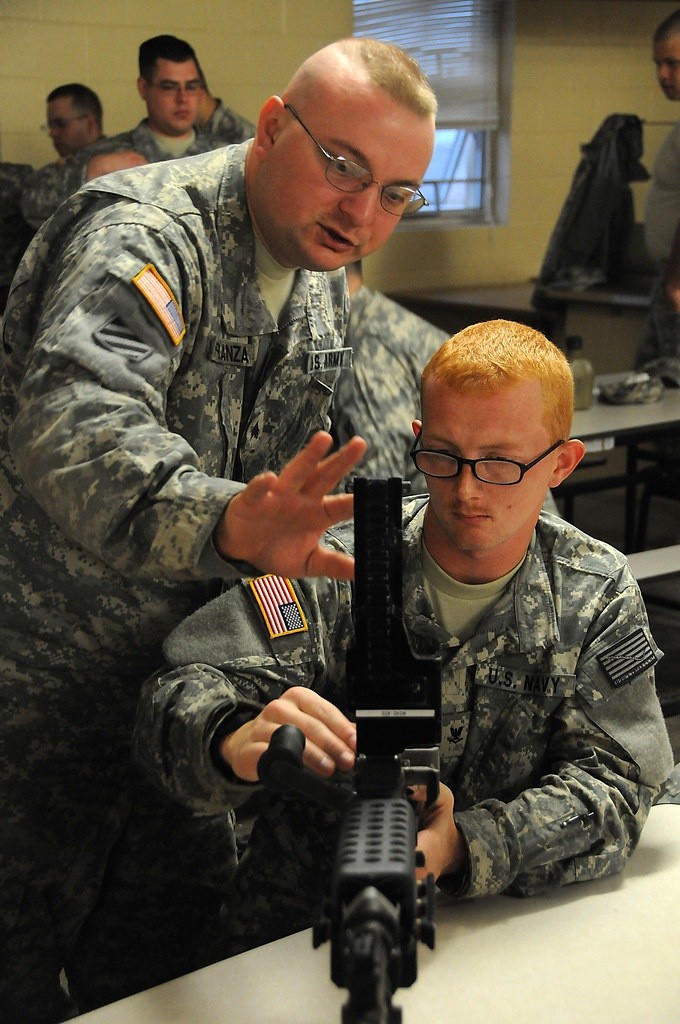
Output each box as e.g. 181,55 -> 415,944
641,10 -> 679,380
125,319 -> 675,927
0,37 -> 455,1023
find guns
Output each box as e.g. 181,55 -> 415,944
221,473 -> 455,1023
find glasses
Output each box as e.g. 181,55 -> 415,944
410,427 -> 564,486
282,102 -> 429,215
143,76 -> 204,97
41,112 -> 90,132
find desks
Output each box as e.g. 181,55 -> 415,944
372,271 -> 680,617
59,803 -> 680,1024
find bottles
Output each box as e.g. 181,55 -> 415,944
563,334 -> 592,412
